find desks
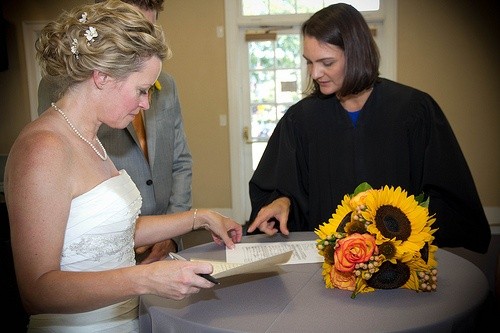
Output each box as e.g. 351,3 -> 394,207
138,231 -> 498,333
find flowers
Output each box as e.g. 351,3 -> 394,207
314,182 -> 439,299
154,78 -> 161,93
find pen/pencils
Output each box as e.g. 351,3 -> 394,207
168,252 -> 221,287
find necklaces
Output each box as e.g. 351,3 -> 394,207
51,102 -> 107,161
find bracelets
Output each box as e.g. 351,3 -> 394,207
192,209 -> 199,231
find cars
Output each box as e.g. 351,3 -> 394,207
251,104 -> 288,141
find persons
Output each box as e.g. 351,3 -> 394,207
247,4 -> 491,254
37,0 -> 193,263
2,0 -> 242,333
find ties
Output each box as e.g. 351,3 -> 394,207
132,109 -> 148,161
